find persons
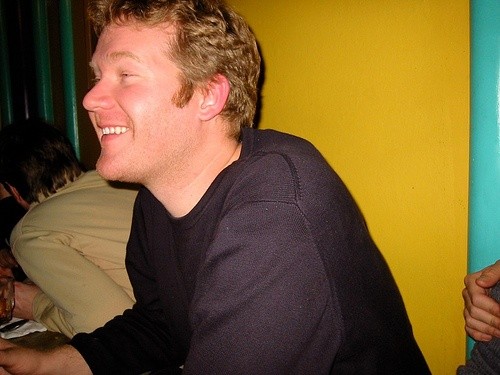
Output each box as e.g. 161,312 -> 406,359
454,257 -> 500,375
0,116 -> 139,335
0,0 -> 430,374
